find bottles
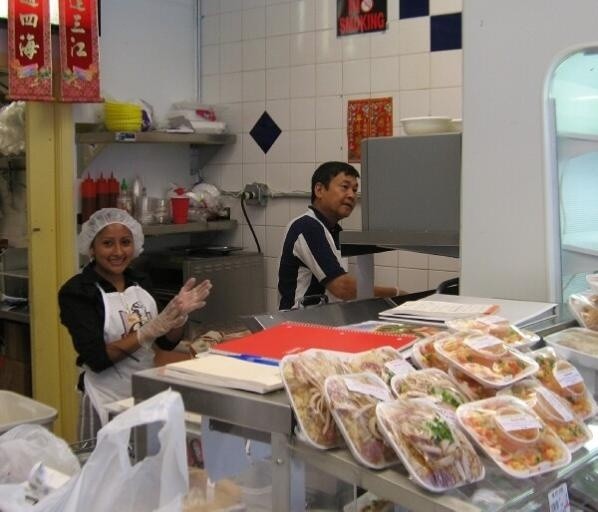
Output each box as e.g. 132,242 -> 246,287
80,169 -> 133,224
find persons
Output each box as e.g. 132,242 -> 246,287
59,208 -> 213,446
274,160 -> 422,313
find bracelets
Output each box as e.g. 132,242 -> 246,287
394,287 -> 399,297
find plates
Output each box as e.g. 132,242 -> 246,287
170,244 -> 246,257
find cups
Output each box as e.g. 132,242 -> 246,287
170,197 -> 190,225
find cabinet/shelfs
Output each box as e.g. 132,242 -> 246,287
75,130 -> 236,237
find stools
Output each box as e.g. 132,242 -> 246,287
79,392 -> 139,466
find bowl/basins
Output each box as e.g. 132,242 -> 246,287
454,118 -> 462,134
400,115 -> 452,136
103,101 -> 144,133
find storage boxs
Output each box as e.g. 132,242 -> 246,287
201,414 -> 307,511
